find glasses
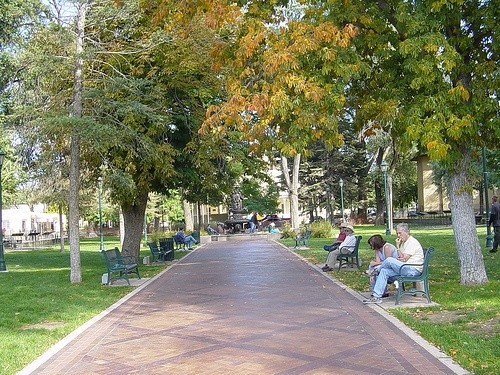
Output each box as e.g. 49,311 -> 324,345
345,230 -> 348,232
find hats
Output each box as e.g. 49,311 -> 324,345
338,223 -> 346,228
345,225 -> 354,233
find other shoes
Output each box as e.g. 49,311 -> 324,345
363,296 -> 383,303
322,265 -> 332,272
381,292 -> 389,298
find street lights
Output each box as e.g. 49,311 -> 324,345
379,160 -> 392,236
326,184 -> 333,220
338,177 -> 345,223
477,122 -> 493,247
96,177 -> 103,252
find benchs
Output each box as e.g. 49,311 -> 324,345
294,229 -> 312,248
328,236 -> 362,273
377,246 -> 434,305
100,247 -> 141,285
146,241 -> 173,265
172,234 -> 191,251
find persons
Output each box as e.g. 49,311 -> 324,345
207,224 -> 220,235
249,212 -> 258,233
322,225 -> 356,272
489,196 -> 500,253
363,222 -> 425,304
368,234 -> 398,298
324,223 -> 349,252
177,227 -> 199,250
